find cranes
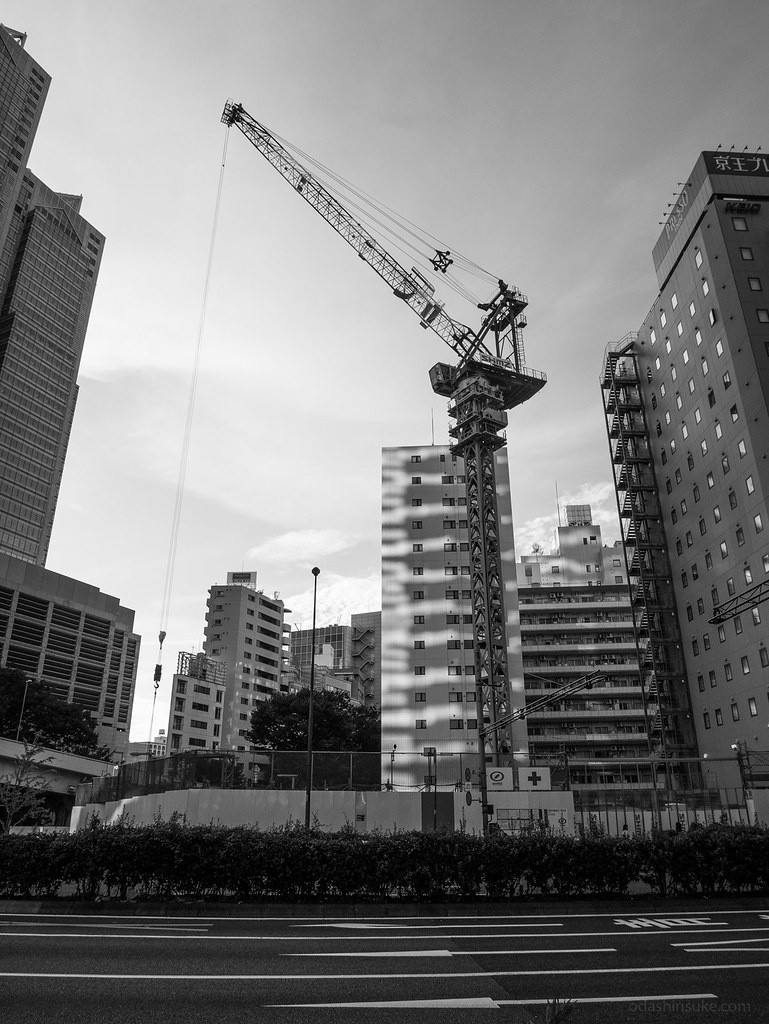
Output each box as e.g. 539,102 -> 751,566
219,103 -> 548,824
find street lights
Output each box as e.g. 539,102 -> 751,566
15,679 -> 33,741
305,566 -> 321,831
731,744 -> 751,828
